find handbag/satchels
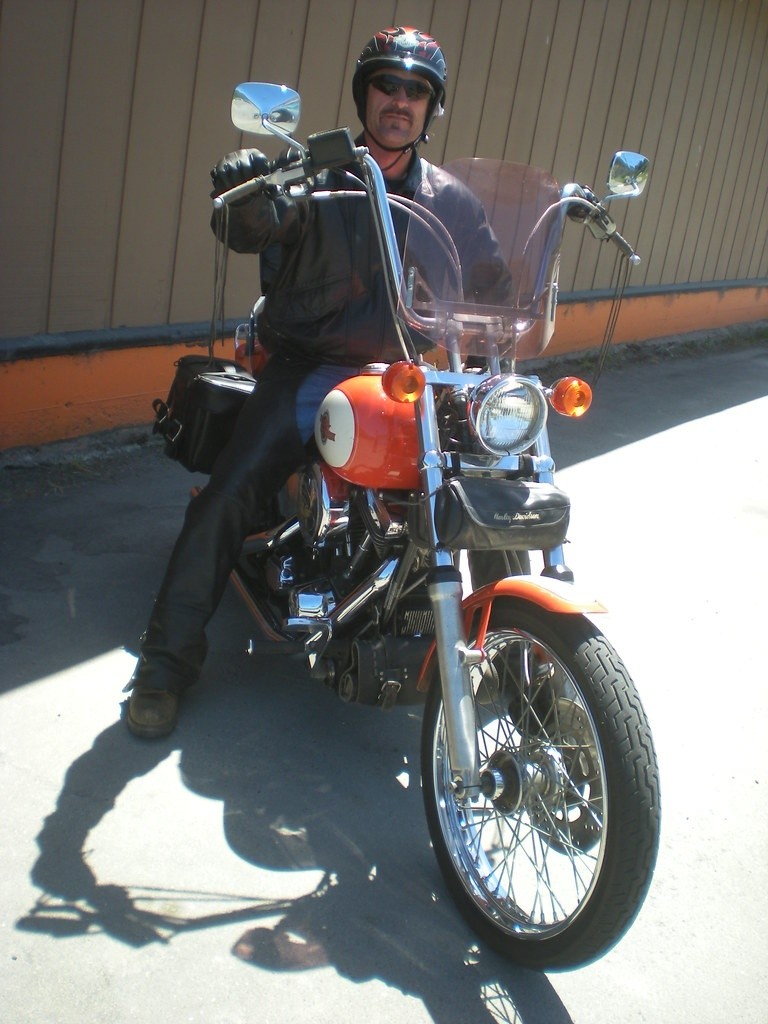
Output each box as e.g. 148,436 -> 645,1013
152,354 -> 258,475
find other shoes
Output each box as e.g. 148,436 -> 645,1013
127,678 -> 181,739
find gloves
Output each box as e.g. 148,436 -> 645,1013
210,147 -> 282,207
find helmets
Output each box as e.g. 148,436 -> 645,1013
352,27 -> 448,126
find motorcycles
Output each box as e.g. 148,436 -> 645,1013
149,81 -> 660,967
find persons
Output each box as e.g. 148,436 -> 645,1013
122,25 -> 562,742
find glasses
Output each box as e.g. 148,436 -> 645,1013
365,75 -> 436,102
